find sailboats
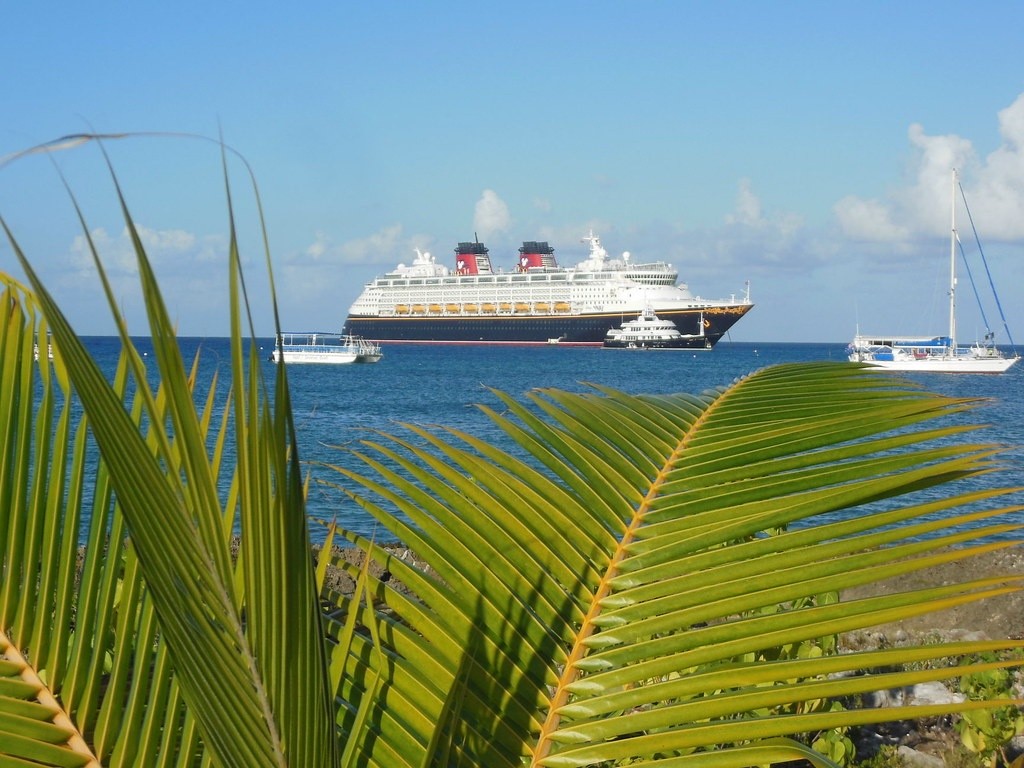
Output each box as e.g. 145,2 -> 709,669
848,168 -> 1022,375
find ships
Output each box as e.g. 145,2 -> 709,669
340,229 -> 755,349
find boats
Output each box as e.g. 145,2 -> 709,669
852,334 -> 952,356
273,330 -> 382,366
600,300 -> 724,349
